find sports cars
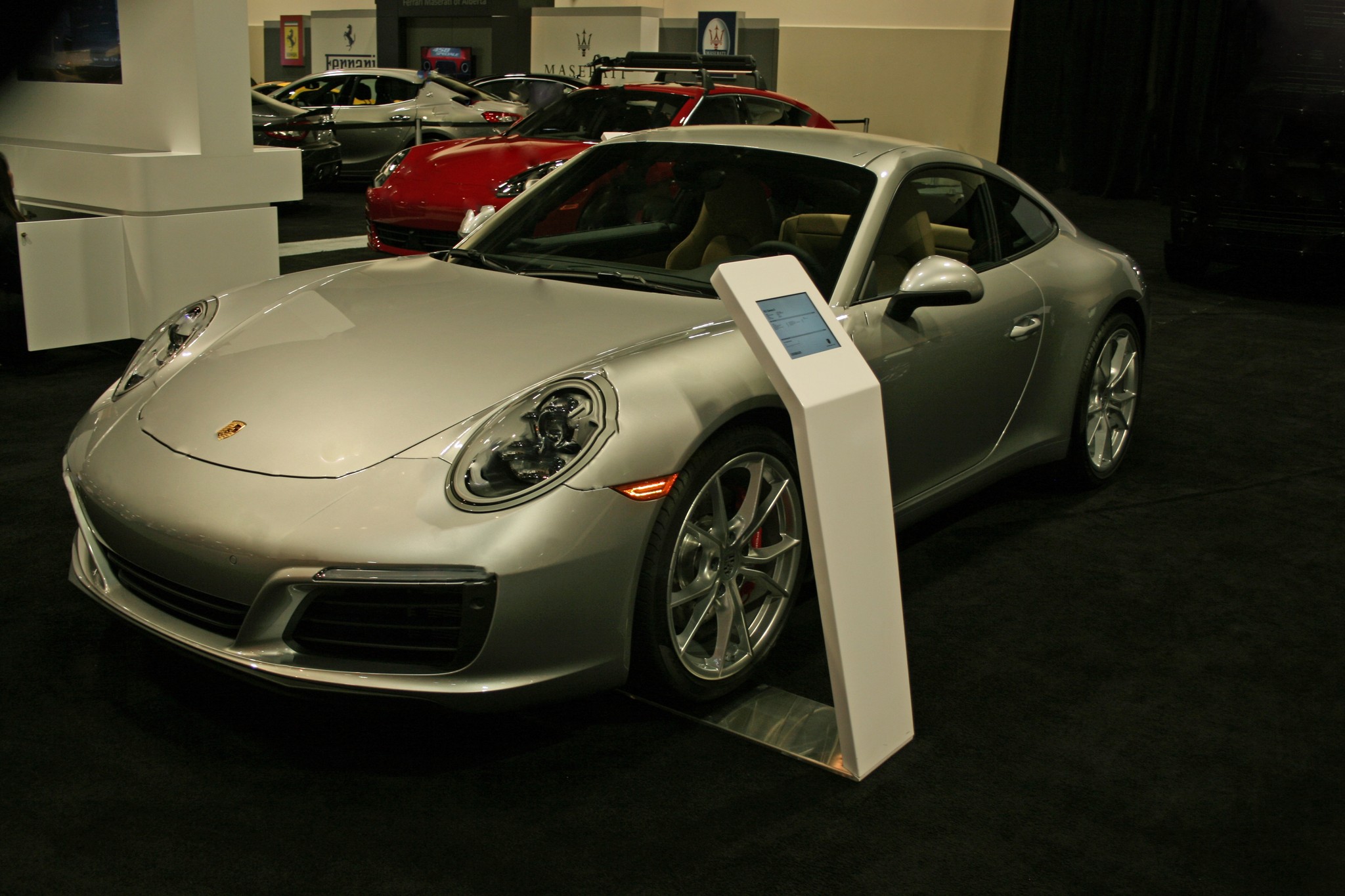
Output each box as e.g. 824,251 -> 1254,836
58,125 -> 1149,718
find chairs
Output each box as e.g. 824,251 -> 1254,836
834,184 -> 936,297
665,180 -> 775,271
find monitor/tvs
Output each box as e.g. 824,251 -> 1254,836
755,292 -> 841,360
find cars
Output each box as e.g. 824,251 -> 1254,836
252,81 -> 403,106
364,51 -> 843,257
251,89 -> 344,182
465,72 -> 589,109
267,67 -> 531,183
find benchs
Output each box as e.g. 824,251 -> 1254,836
776,213 -> 978,266
